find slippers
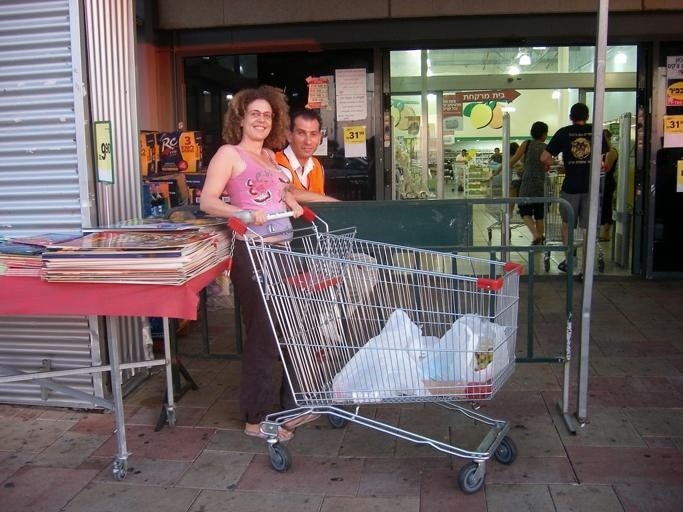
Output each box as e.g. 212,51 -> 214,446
285,406 -> 325,431
243,420 -> 294,442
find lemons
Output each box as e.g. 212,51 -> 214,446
391,104 -> 415,131
471,104 -> 504,128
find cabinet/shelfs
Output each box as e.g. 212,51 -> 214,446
463,165 -> 492,200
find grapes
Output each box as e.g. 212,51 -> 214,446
475,348 -> 494,369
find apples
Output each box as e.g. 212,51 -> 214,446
176,159 -> 188,171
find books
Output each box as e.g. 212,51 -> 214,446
0,216 -> 235,286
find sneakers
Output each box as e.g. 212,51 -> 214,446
558,259 -> 570,273
530,238 -> 541,248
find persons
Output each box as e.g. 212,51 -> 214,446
636,122 -> 643,173
489,142 -> 525,219
539,102 -> 610,283
455,149 -> 472,192
199,87 -> 322,442
510,120 -> 549,245
597,128 -> 619,243
483,147 -> 502,164
274,108 -> 342,204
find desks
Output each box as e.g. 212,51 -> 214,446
1,257 -> 232,479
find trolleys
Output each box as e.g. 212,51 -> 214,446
227,207 -> 525,494
487,172 -> 605,272
